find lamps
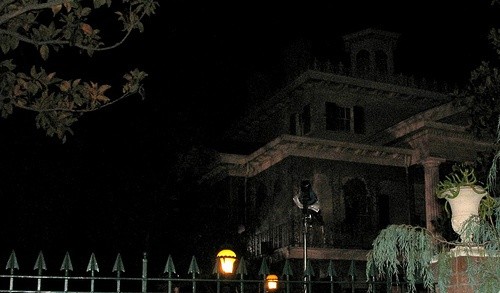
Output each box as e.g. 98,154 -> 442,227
266,274 -> 278,291
298,179 -> 317,292
217,249 -> 238,277
